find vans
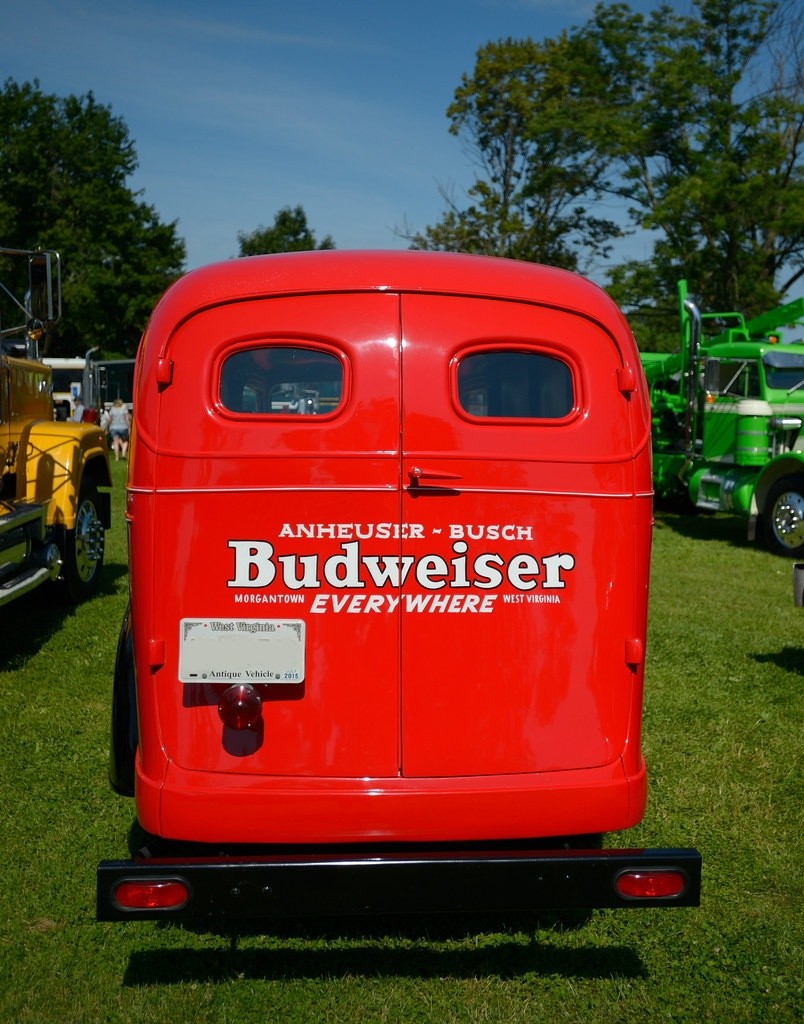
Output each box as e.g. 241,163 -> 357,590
96,250 -> 701,921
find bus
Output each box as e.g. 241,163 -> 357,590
42,357 -> 135,421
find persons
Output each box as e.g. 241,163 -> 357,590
81,404 -> 96,424
72,398 -> 83,421
99,399 -> 132,462
55,400 -> 67,421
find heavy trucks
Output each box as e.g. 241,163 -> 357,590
0,248 -> 112,604
639,279 -> 804,558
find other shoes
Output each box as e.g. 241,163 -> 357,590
121,456 -> 127,460
